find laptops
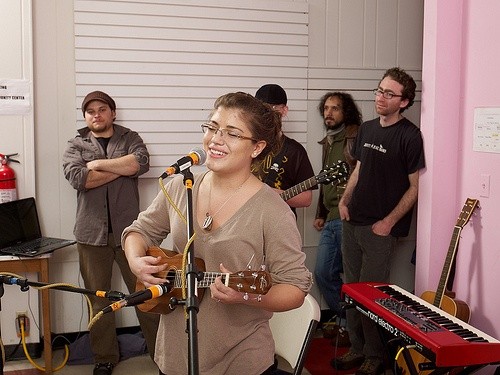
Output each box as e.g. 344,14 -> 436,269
0,197 -> 77,257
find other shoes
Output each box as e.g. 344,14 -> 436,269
331,350 -> 365,370
355,358 -> 386,375
93,361 -> 113,375
323,324 -> 352,347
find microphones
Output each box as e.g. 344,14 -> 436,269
159,147 -> 207,179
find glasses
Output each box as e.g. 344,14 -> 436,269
201,122 -> 257,143
373,89 -> 403,99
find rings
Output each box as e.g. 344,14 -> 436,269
216,300 -> 221,303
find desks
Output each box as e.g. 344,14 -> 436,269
0,256 -> 53,375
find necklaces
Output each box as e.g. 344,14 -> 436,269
201,170 -> 251,232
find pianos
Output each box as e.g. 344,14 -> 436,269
339,280 -> 500,375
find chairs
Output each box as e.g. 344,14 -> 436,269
269,293 -> 321,375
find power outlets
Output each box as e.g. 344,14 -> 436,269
15,311 -> 30,337
480,173 -> 491,198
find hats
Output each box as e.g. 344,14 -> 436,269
81,91 -> 116,112
255,84 -> 287,106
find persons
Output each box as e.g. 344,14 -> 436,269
251,84 -> 319,221
331,66 -> 427,375
313,92 -> 363,309
120,92 -> 313,375
61,91 -> 160,375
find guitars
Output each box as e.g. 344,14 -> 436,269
279,159 -> 351,203
135,247 -> 272,315
396,198 -> 481,375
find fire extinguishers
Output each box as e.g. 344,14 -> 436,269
0,152 -> 21,204
